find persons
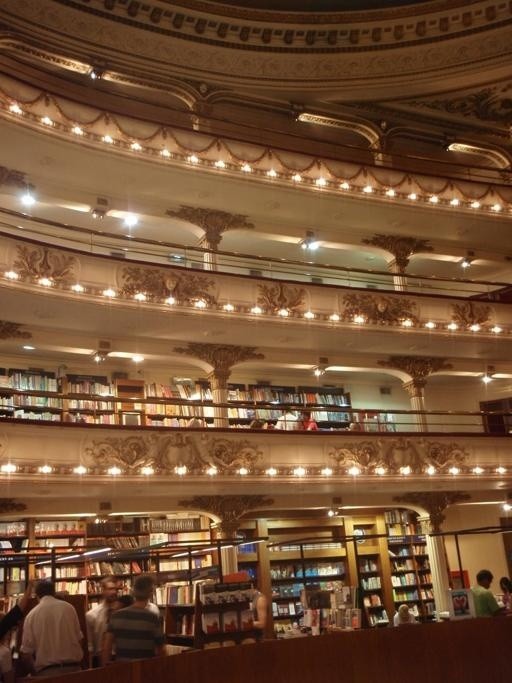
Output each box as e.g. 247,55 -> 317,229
272,407 -> 318,432
21,578 -> 84,675
2,646 -> 22,682
470,569 -> 512,616
81,577 -> 126,668
102,575 -> 167,666
394,605 -> 416,627
497,576 -> 512,612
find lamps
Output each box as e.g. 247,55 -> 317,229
89,349 -> 110,363
479,366 -> 496,384
299,230 -> 322,251
312,361 -> 327,378
458,256 -> 474,270
86,62 -> 107,81
20,180 -> 36,206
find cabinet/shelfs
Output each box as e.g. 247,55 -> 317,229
0,366 -> 352,430
0,504 -> 438,653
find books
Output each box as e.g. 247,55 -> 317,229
146,382 -> 214,427
227,389 -> 351,422
384,509 -> 436,616
0,373 -> 115,424
270,561 -> 347,632
1,507 -> 255,636
358,555 -> 388,626
